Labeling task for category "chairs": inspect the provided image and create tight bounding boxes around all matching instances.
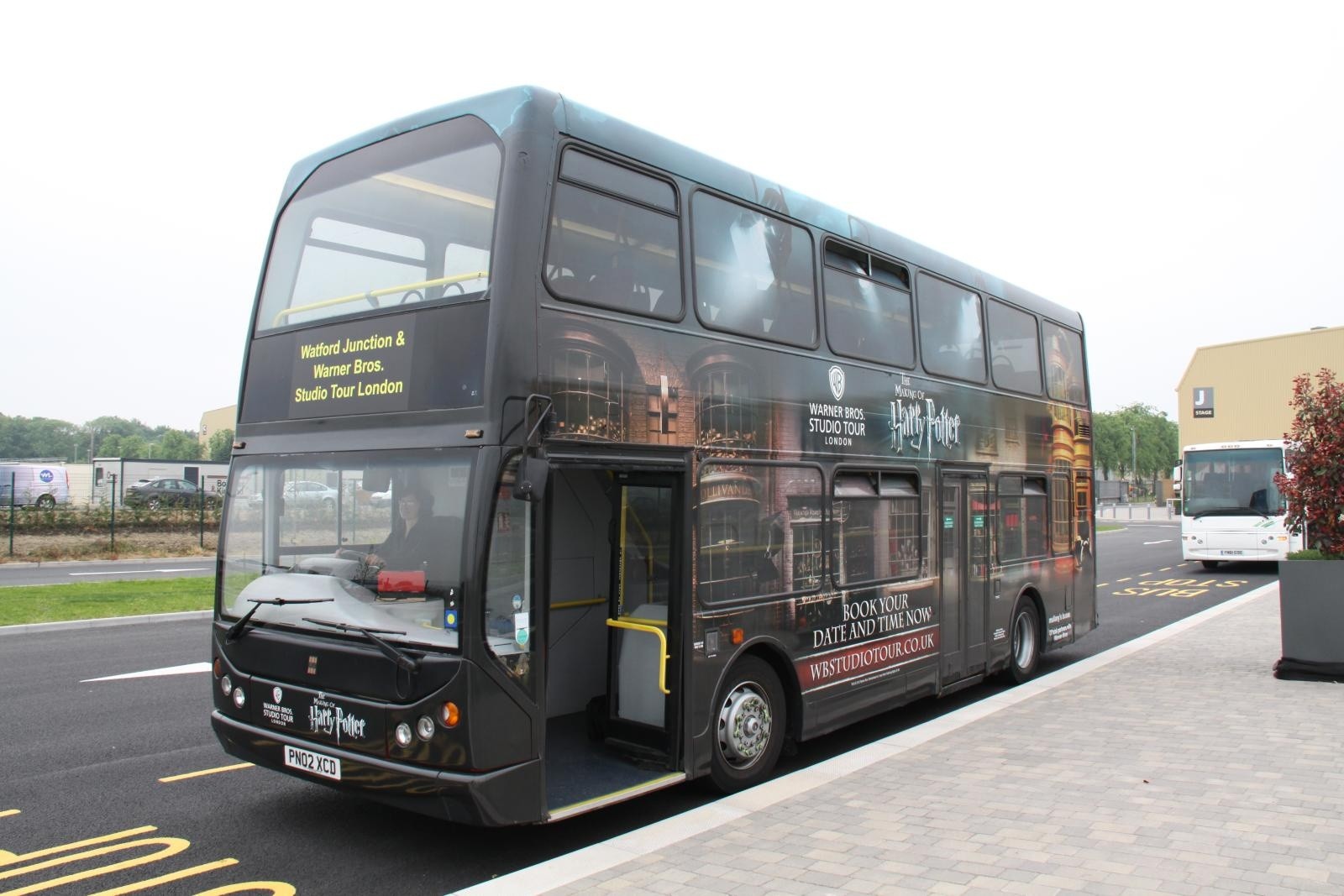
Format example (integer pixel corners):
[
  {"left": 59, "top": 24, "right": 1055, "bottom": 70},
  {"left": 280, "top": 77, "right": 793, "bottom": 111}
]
[{"left": 548, "top": 261, "right": 1085, "bottom": 401}]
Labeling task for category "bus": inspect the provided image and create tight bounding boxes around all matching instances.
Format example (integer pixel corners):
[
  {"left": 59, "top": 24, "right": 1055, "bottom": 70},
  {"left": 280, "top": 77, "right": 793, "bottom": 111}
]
[
  {"left": 212, "top": 86, "right": 1098, "bottom": 825},
  {"left": 1173, "top": 440, "right": 1310, "bottom": 570}
]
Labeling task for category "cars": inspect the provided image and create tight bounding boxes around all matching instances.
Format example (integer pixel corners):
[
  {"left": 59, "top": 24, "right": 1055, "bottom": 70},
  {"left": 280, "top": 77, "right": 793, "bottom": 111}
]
[
  {"left": 250, "top": 480, "right": 346, "bottom": 510},
  {"left": 124, "top": 477, "right": 225, "bottom": 511}
]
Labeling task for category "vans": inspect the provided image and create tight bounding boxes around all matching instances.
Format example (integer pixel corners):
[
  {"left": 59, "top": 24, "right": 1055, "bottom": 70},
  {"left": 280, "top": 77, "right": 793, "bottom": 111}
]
[{"left": 0, "top": 462, "right": 70, "bottom": 511}]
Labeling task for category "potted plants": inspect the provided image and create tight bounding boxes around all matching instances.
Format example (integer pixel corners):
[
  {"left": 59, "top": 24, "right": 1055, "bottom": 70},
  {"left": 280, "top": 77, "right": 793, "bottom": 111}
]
[{"left": 1271, "top": 548, "right": 1344, "bottom": 683}]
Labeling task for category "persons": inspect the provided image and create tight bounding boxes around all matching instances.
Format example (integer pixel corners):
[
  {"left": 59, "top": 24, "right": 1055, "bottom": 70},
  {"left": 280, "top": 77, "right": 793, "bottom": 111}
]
[{"left": 333, "top": 482, "right": 450, "bottom": 572}]
